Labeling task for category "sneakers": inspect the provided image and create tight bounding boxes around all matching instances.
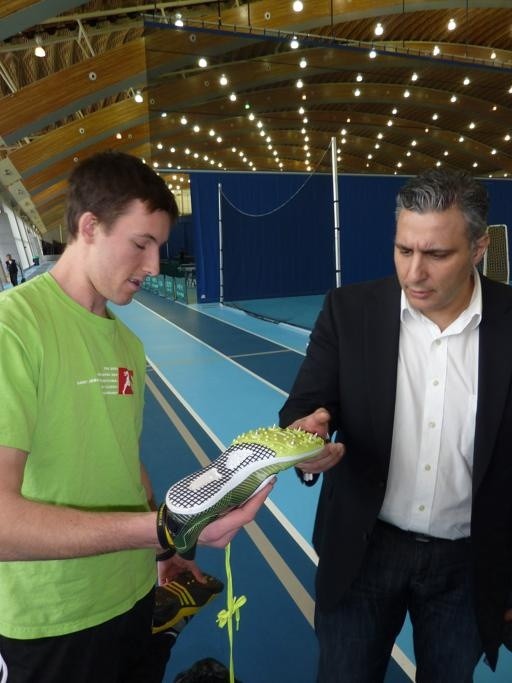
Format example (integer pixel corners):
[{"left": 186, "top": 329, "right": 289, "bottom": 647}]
[
  {"left": 153, "top": 571, "right": 223, "bottom": 633},
  {"left": 162, "top": 427, "right": 324, "bottom": 560}
]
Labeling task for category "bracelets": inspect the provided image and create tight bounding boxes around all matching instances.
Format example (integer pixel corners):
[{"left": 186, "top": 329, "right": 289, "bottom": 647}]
[{"left": 153, "top": 499, "right": 176, "bottom": 550}]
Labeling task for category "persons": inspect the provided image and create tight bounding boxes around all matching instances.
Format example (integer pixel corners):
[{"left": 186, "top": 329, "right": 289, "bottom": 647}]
[
  {"left": 272, "top": 165, "right": 510, "bottom": 682},
  {"left": 0, "top": 142, "right": 280, "bottom": 681},
  {"left": 3, "top": 253, "right": 21, "bottom": 288}
]
